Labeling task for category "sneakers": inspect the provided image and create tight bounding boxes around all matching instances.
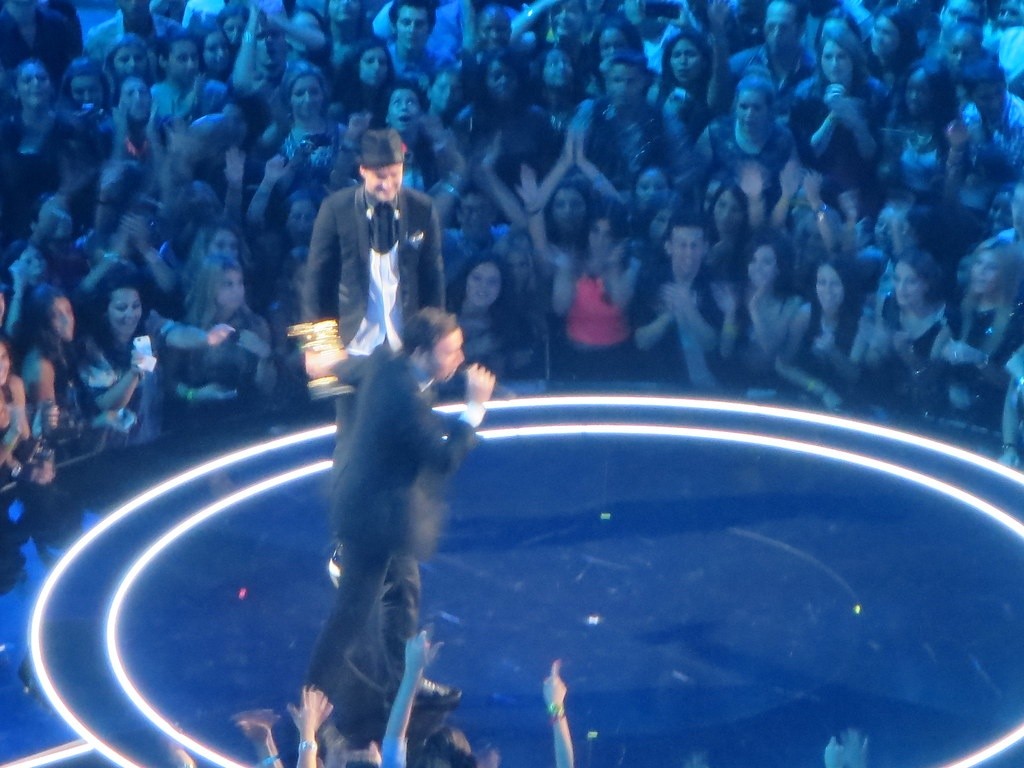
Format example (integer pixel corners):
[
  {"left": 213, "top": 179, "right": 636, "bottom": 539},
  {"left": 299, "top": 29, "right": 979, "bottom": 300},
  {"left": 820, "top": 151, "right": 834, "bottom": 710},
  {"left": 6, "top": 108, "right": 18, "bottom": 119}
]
[
  {"left": 383, "top": 678, "right": 464, "bottom": 711},
  {"left": 330, "top": 542, "right": 343, "bottom": 575}
]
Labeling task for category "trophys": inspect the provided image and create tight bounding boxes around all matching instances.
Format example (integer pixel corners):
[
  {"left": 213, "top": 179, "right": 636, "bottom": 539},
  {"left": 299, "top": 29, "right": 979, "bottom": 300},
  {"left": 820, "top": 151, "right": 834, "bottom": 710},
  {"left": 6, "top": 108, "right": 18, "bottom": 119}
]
[{"left": 285, "top": 318, "right": 353, "bottom": 401}]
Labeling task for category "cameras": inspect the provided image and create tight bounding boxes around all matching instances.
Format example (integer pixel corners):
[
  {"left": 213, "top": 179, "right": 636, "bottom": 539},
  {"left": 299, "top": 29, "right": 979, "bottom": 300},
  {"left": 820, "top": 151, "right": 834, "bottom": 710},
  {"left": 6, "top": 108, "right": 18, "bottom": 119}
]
[{"left": 27, "top": 439, "right": 53, "bottom": 464}]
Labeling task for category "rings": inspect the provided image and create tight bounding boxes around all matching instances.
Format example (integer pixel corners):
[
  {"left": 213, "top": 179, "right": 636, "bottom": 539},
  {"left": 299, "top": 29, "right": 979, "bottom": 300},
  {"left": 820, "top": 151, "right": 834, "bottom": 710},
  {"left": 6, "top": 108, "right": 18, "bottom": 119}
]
[{"left": 955, "top": 352, "right": 957, "bottom": 357}]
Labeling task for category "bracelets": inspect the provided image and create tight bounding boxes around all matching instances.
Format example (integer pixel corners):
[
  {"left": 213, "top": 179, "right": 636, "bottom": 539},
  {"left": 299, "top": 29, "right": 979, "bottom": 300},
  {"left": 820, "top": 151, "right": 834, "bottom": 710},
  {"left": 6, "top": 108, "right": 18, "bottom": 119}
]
[
  {"left": 526, "top": 208, "right": 542, "bottom": 216},
  {"left": 551, "top": 714, "right": 565, "bottom": 721},
  {"left": 299, "top": 741, "right": 317, "bottom": 751},
  {"left": 186, "top": 388, "right": 195, "bottom": 400},
  {"left": 976, "top": 354, "right": 989, "bottom": 369},
  {"left": 723, "top": 324, "right": 737, "bottom": 335},
  {"left": 262, "top": 754, "right": 280, "bottom": 768},
  {"left": 1001, "top": 442, "right": 1017, "bottom": 449},
  {"left": 813, "top": 204, "right": 828, "bottom": 221},
  {"left": 807, "top": 380, "right": 816, "bottom": 392}
]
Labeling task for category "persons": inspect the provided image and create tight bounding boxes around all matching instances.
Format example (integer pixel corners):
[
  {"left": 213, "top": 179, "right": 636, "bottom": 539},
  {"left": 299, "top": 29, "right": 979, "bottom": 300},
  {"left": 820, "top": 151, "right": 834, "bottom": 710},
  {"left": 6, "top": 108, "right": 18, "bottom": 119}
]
[
  {"left": 302, "top": 126, "right": 444, "bottom": 586},
  {"left": 235, "top": 630, "right": 574, "bottom": 768},
  {"left": 824, "top": 728, "right": 869, "bottom": 768},
  {"left": 0, "top": 0, "right": 1024, "bottom": 485},
  {"left": 308, "top": 307, "right": 497, "bottom": 723}
]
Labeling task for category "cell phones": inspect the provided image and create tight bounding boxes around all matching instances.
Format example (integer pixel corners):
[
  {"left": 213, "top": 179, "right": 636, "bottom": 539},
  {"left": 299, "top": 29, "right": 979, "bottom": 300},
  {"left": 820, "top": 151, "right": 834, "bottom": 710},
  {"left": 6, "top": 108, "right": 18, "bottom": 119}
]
[
  {"left": 308, "top": 132, "right": 334, "bottom": 146},
  {"left": 132, "top": 334, "right": 152, "bottom": 357}
]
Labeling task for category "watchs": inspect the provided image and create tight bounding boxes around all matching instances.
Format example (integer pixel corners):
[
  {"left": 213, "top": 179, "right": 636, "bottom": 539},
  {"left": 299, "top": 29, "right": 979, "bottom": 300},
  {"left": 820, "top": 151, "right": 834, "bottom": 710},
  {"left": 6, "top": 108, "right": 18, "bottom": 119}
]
[{"left": 548, "top": 704, "right": 563, "bottom": 714}]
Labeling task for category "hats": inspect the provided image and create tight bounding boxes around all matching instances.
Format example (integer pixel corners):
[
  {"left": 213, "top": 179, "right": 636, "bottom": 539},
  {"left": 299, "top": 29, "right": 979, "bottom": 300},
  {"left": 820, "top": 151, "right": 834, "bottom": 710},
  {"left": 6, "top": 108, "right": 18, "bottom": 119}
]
[{"left": 355, "top": 128, "right": 406, "bottom": 169}]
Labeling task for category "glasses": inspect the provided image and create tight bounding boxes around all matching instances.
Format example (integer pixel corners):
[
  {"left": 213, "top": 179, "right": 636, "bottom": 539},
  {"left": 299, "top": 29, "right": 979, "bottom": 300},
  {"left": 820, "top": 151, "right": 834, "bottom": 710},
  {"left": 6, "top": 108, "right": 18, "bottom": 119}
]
[{"left": 256, "top": 29, "right": 281, "bottom": 41}]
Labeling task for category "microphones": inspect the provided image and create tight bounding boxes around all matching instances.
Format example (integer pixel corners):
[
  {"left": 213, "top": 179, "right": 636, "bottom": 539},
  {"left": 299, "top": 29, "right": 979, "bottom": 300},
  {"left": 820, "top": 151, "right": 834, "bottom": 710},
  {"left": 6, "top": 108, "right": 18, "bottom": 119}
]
[{"left": 460, "top": 365, "right": 512, "bottom": 399}]
